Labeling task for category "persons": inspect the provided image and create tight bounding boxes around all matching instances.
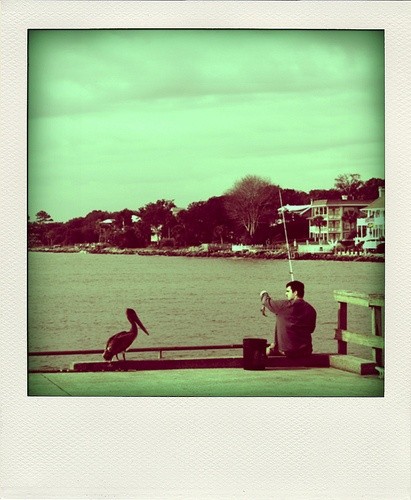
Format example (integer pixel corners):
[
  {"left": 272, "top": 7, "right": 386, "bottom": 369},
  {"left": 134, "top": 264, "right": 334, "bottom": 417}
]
[{"left": 259, "top": 281, "right": 317, "bottom": 356}]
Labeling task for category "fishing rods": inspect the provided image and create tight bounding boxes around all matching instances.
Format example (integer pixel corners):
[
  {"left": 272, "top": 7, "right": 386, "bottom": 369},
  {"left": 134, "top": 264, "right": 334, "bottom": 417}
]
[{"left": 279, "top": 188, "right": 294, "bottom": 282}]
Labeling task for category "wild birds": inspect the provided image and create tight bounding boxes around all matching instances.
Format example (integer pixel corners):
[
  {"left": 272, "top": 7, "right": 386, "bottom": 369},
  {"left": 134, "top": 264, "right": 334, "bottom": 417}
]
[{"left": 103, "top": 308, "right": 150, "bottom": 372}]
